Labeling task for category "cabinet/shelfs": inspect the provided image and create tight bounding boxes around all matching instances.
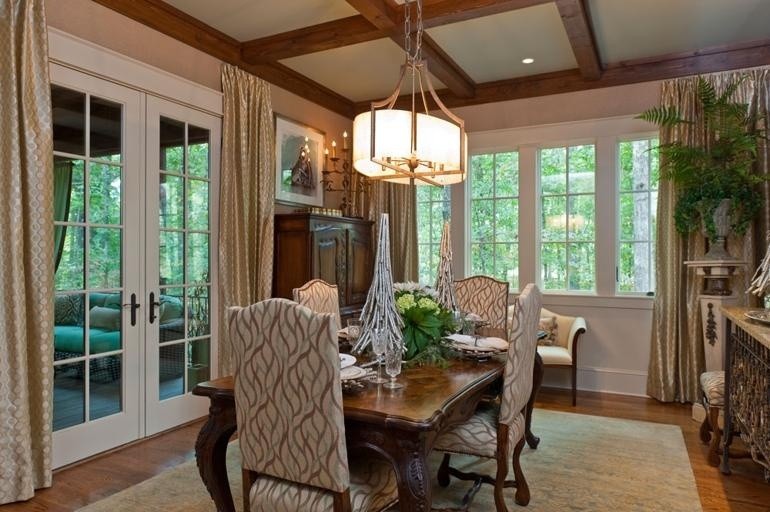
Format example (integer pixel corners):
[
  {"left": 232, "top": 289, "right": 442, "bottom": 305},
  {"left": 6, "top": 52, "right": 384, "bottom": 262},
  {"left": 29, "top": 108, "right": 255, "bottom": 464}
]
[{"left": 269, "top": 214, "right": 375, "bottom": 325}]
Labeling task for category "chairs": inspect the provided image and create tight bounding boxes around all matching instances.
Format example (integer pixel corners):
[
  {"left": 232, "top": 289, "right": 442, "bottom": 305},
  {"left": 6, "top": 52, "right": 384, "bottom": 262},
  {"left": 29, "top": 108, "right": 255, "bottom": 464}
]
[
  {"left": 223, "top": 297, "right": 400, "bottom": 512},
  {"left": 429, "top": 282, "right": 542, "bottom": 512},
  {"left": 446, "top": 273, "right": 510, "bottom": 343},
  {"left": 507, "top": 304, "right": 588, "bottom": 408},
  {"left": 292, "top": 276, "right": 341, "bottom": 350}
]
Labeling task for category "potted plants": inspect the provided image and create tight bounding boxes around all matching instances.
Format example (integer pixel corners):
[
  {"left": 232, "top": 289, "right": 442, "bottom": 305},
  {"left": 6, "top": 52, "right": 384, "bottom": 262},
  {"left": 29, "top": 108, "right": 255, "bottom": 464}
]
[{"left": 630, "top": 70, "right": 770, "bottom": 264}]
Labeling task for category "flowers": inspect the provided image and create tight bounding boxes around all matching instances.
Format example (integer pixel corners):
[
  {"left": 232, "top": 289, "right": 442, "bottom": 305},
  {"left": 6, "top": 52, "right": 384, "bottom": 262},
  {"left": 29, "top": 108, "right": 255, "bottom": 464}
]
[{"left": 391, "top": 275, "right": 450, "bottom": 357}]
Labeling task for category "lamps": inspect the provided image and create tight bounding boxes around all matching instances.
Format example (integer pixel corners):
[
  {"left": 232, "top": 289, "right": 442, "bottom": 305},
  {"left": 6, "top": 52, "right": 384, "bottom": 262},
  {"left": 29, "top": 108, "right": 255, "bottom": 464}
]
[
  {"left": 320, "top": 129, "right": 369, "bottom": 217},
  {"left": 351, "top": 0, "right": 470, "bottom": 189}
]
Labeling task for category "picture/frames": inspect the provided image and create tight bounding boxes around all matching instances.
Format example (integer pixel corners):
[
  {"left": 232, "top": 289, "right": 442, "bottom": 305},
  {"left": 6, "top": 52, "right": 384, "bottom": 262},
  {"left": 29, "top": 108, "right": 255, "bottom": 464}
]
[{"left": 274, "top": 111, "right": 326, "bottom": 212}]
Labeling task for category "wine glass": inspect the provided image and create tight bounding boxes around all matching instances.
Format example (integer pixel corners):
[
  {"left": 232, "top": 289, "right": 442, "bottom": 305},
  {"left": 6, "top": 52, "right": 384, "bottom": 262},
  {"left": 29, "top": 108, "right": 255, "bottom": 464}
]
[
  {"left": 370, "top": 328, "right": 390, "bottom": 384},
  {"left": 384, "top": 350, "right": 402, "bottom": 391},
  {"left": 449, "top": 310, "right": 464, "bottom": 342},
  {"left": 347, "top": 317, "right": 362, "bottom": 363}
]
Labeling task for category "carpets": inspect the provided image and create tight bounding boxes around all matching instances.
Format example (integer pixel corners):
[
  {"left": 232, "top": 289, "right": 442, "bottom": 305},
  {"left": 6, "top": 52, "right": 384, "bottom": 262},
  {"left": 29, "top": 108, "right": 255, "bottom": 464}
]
[{"left": 72, "top": 406, "right": 708, "bottom": 512}]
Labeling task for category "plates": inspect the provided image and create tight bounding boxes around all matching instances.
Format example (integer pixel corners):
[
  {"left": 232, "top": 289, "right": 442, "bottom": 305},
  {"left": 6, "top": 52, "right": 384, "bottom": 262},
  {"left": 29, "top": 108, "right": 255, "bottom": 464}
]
[
  {"left": 339, "top": 353, "right": 354, "bottom": 370},
  {"left": 340, "top": 366, "right": 365, "bottom": 380}
]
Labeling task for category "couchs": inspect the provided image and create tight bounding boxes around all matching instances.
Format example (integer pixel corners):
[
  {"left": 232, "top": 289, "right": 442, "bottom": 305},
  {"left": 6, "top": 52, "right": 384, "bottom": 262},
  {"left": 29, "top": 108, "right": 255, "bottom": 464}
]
[{"left": 49, "top": 289, "right": 195, "bottom": 391}]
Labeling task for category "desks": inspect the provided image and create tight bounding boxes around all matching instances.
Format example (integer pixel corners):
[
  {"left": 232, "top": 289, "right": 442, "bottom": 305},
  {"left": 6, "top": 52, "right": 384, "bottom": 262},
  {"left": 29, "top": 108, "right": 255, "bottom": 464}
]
[{"left": 720, "top": 305, "right": 770, "bottom": 481}]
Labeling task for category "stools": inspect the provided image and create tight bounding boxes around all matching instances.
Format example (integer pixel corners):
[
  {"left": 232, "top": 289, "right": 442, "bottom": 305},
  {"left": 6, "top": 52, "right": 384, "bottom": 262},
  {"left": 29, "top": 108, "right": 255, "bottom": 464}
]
[{"left": 697, "top": 370, "right": 727, "bottom": 466}]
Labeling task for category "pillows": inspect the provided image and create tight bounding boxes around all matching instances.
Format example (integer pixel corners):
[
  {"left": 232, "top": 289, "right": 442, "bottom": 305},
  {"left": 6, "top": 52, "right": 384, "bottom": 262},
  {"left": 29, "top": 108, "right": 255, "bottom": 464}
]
[
  {"left": 55, "top": 290, "right": 83, "bottom": 327},
  {"left": 538, "top": 314, "right": 557, "bottom": 346},
  {"left": 88, "top": 305, "right": 124, "bottom": 333}
]
